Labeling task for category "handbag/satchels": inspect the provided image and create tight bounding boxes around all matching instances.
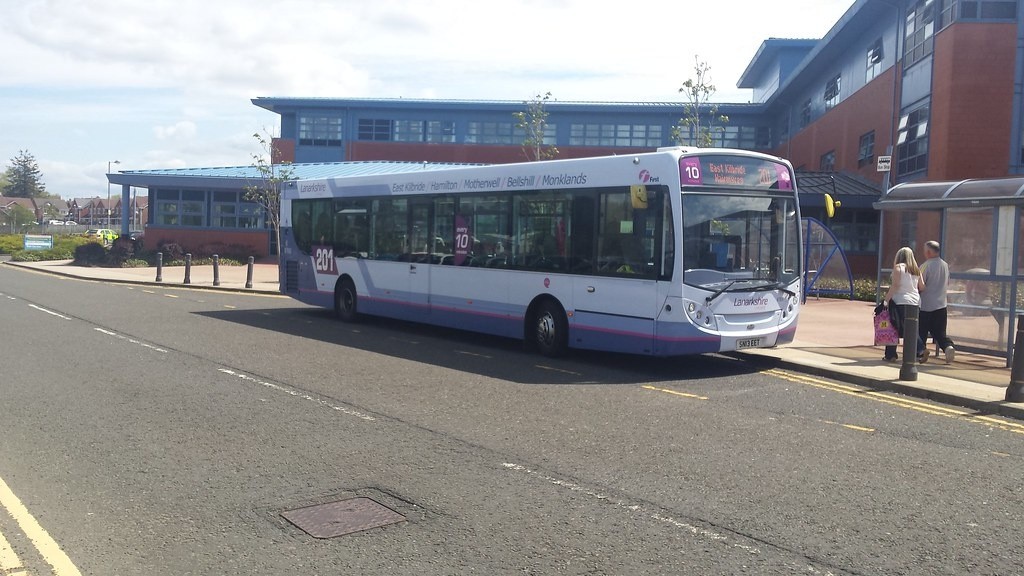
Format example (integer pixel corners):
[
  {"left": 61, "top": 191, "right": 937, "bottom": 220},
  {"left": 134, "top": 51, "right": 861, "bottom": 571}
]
[{"left": 874, "top": 309, "right": 899, "bottom": 346}]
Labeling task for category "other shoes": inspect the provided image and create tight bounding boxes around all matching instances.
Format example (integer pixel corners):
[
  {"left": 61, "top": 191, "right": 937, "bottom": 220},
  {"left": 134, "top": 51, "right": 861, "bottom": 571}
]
[
  {"left": 882, "top": 356, "right": 896, "bottom": 362},
  {"left": 945, "top": 346, "right": 954, "bottom": 363},
  {"left": 919, "top": 348, "right": 930, "bottom": 364}
]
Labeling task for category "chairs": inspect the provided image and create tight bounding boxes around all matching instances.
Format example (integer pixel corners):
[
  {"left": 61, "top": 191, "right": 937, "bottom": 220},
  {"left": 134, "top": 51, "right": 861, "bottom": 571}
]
[{"left": 397, "top": 249, "right": 528, "bottom": 269}]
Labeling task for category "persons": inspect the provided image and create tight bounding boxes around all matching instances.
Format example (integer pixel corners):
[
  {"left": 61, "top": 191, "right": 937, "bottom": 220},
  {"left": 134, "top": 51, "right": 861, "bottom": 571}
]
[
  {"left": 882, "top": 246, "right": 929, "bottom": 364},
  {"left": 918, "top": 240, "right": 955, "bottom": 363}
]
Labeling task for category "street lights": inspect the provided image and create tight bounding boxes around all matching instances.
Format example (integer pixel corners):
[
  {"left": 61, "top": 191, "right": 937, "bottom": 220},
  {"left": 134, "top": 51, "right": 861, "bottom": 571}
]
[{"left": 108, "top": 160, "right": 121, "bottom": 229}]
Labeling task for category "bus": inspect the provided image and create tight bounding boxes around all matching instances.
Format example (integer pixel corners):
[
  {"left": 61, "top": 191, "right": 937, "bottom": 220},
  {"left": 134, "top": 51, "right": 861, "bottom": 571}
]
[{"left": 278, "top": 145, "right": 841, "bottom": 358}]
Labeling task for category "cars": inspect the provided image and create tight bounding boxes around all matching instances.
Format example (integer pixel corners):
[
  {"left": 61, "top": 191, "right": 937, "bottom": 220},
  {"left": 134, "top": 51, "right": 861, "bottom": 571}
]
[
  {"left": 50, "top": 220, "right": 64, "bottom": 225},
  {"left": 84, "top": 229, "right": 118, "bottom": 245},
  {"left": 66, "top": 221, "right": 77, "bottom": 225}
]
